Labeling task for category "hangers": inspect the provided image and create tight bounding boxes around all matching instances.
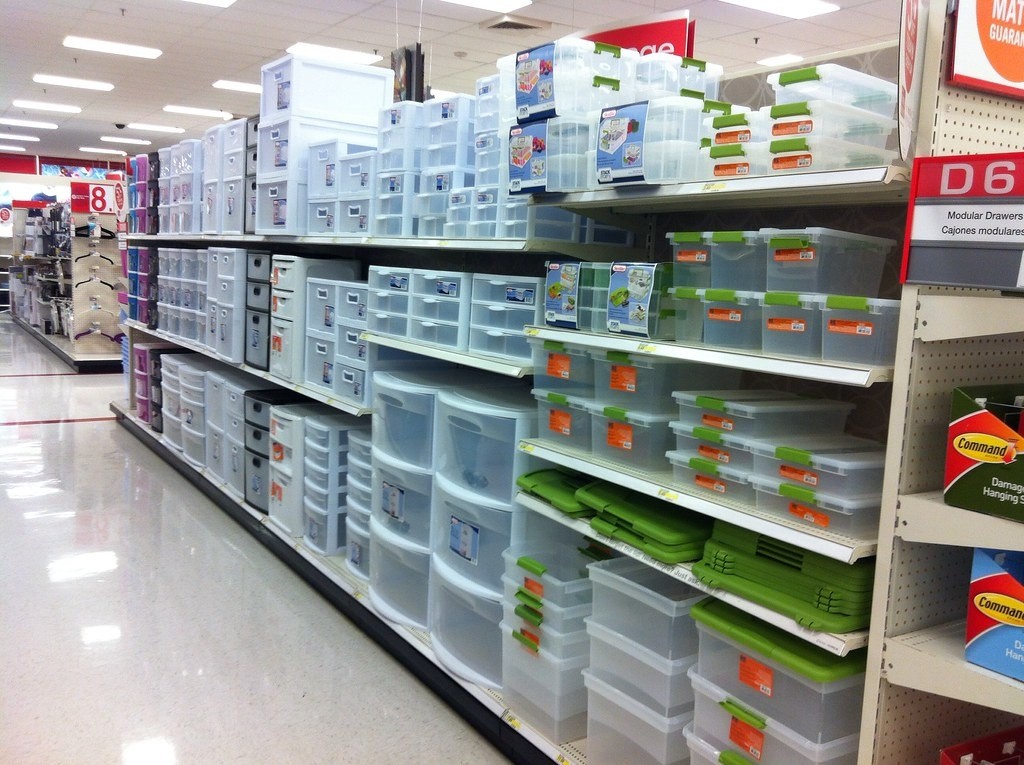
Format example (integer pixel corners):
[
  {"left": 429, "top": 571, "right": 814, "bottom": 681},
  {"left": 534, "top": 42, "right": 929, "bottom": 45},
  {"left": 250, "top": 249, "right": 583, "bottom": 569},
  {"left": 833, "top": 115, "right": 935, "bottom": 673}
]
[
  {"left": 75, "top": 269, "right": 115, "bottom": 291},
  {"left": 79, "top": 298, "right": 115, "bottom": 317},
  {"left": 75, "top": 325, "right": 115, "bottom": 342},
  {"left": 68, "top": 225, "right": 115, "bottom": 240},
  {"left": 75, "top": 254, "right": 114, "bottom": 264}
]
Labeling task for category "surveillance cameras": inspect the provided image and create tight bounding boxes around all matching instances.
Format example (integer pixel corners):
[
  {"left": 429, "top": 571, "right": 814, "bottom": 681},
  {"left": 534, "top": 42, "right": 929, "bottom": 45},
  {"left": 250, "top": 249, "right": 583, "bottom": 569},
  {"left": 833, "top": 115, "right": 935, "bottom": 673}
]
[{"left": 115, "top": 124, "right": 125, "bottom": 130}]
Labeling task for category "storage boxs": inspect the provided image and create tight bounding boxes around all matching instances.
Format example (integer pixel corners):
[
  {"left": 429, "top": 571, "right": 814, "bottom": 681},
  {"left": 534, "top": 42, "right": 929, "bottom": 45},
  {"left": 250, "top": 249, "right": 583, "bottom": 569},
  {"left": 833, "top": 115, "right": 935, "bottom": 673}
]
[
  {"left": 121, "top": 37, "right": 899, "bottom": 765},
  {"left": 965, "top": 548, "right": 1024, "bottom": 683},
  {"left": 943, "top": 382, "right": 1024, "bottom": 523},
  {"left": 939, "top": 726, "right": 1024, "bottom": 765}
]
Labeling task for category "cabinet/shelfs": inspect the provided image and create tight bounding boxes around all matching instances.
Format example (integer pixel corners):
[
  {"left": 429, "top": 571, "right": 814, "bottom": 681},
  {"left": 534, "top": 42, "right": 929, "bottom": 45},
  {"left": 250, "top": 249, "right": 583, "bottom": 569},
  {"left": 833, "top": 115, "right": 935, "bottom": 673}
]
[
  {"left": 9, "top": 208, "right": 123, "bottom": 373},
  {"left": 858, "top": 278, "right": 1024, "bottom": 765},
  {"left": 517, "top": 166, "right": 909, "bottom": 657},
  {"left": 111, "top": 232, "right": 654, "bottom": 765}
]
